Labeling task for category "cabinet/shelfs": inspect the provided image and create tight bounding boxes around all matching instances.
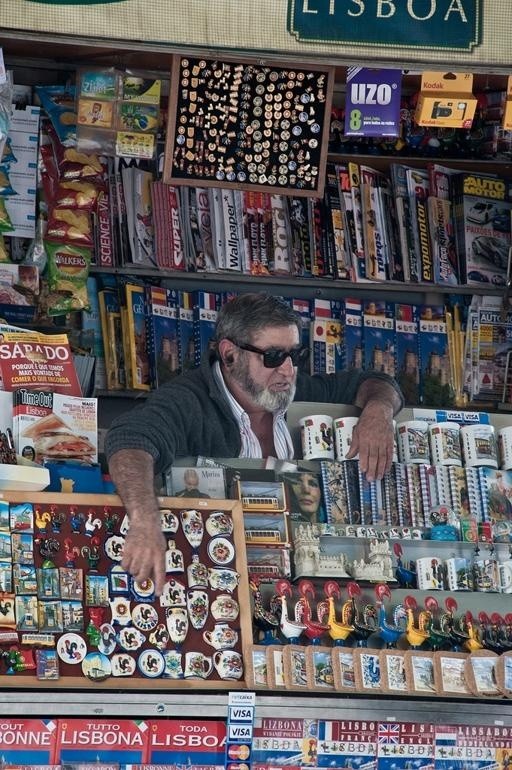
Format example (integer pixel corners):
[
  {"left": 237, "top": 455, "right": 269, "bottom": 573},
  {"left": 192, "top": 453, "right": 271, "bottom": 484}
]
[
  {"left": 0, "top": 29, "right": 512, "bottom": 413},
  {"left": 0, "top": 691, "right": 512, "bottom": 770}
]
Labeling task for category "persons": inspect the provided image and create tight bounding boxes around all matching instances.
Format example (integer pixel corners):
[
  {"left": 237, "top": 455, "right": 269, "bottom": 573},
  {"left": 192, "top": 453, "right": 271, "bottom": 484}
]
[{"left": 104, "top": 292, "right": 406, "bottom": 597}]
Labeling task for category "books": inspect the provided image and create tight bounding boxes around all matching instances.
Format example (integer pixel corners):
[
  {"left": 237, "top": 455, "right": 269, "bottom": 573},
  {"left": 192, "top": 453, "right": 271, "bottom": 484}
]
[
  {"left": 98, "top": 290, "right": 125, "bottom": 390},
  {"left": 193, "top": 290, "right": 221, "bottom": 368},
  {"left": 0, "top": 717, "right": 510, "bottom": 764},
  {"left": 285, "top": 296, "right": 311, "bottom": 347},
  {"left": 95, "top": 155, "right": 512, "bottom": 285},
  {"left": 144, "top": 287, "right": 179, "bottom": 390},
  {"left": 224, "top": 291, "right": 242, "bottom": 304},
  {"left": 390, "top": 303, "right": 420, "bottom": 381},
  {"left": 361, "top": 299, "right": 395, "bottom": 376},
  {"left": 118, "top": 285, "right": 151, "bottom": 391},
  {"left": 450, "top": 294, "right": 512, "bottom": 404},
  {"left": 320, "top": 458, "right": 512, "bottom": 529},
  {"left": 309, "top": 297, "right": 344, "bottom": 376},
  {"left": 82, "top": 277, "right": 105, "bottom": 389},
  {"left": 14, "top": 391, "right": 96, "bottom": 466},
  {"left": 176, "top": 290, "right": 194, "bottom": 375},
  {"left": 341, "top": 297, "right": 363, "bottom": 370},
  {"left": 417, "top": 305, "right": 448, "bottom": 405},
  {"left": 0, "top": 331, "right": 83, "bottom": 398}
]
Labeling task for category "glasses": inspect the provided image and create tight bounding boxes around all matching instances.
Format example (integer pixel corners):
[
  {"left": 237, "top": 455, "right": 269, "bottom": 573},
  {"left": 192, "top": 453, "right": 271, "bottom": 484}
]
[{"left": 233, "top": 340, "right": 310, "bottom": 366}]
